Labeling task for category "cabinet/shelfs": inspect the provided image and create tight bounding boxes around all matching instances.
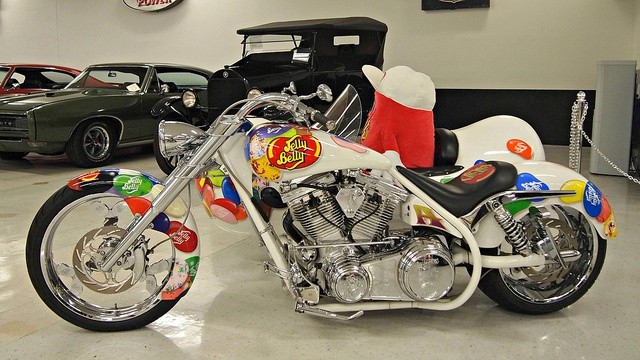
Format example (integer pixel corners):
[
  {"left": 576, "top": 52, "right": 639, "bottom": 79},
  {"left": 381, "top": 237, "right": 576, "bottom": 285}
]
[{"left": 589, "top": 58, "right": 635, "bottom": 176}]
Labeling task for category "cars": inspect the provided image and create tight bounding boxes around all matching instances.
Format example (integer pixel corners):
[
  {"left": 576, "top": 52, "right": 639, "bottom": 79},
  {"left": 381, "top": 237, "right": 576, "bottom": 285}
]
[
  {"left": 0, "top": 62, "right": 214, "bottom": 168},
  {"left": 0, "top": 64, "right": 122, "bottom": 155}
]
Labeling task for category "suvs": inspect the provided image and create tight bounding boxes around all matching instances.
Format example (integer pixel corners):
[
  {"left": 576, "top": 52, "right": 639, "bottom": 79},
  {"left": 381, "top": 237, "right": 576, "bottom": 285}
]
[{"left": 150, "top": 17, "right": 388, "bottom": 175}]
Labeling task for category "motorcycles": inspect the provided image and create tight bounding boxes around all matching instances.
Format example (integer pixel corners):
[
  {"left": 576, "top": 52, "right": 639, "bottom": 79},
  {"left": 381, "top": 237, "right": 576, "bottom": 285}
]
[{"left": 25, "top": 81, "right": 618, "bottom": 332}]
